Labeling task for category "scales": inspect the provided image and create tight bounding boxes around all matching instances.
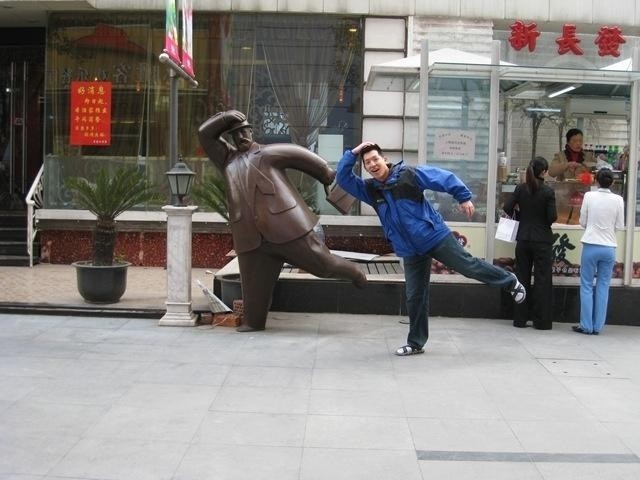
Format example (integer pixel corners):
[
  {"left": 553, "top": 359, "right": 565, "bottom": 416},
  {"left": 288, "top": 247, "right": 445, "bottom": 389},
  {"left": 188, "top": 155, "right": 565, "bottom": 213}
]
[{"left": 595, "top": 170, "right": 623, "bottom": 179}]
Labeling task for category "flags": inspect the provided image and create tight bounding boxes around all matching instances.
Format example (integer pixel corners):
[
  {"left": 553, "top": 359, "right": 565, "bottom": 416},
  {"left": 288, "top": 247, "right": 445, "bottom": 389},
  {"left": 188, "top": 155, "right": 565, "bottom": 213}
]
[
  {"left": 180, "top": 0, "right": 196, "bottom": 79},
  {"left": 158, "top": 0, "right": 182, "bottom": 64}
]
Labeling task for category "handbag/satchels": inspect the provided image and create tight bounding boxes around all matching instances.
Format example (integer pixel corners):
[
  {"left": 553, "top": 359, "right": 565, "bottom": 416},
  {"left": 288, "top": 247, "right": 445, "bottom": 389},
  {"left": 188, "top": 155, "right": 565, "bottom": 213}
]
[{"left": 494, "top": 208, "right": 520, "bottom": 244}]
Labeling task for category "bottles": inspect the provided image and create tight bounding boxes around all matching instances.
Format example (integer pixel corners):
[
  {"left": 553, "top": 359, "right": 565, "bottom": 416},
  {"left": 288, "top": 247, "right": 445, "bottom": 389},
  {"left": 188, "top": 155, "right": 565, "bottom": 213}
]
[
  {"left": 497, "top": 152, "right": 507, "bottom": 182},
  {"left": 584, "top": 143, "right": 619, "bottom": 169}
]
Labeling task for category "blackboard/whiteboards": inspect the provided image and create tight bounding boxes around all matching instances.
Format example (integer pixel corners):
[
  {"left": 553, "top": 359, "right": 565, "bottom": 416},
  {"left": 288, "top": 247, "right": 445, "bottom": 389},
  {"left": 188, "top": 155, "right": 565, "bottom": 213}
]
[{"left": 69, "top": 81, "right": 113, "bottom": 146}]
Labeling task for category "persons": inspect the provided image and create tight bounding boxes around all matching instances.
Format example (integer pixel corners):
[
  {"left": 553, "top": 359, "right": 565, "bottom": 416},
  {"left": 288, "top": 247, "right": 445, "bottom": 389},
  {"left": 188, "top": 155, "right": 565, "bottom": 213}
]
[
  {"left": 595, "top": 144, "right": 631, "bottom": 195},
  {"left": 505, "top": 155, "right": 555, "bottom": 332},
  {"left": 570, "top": 167, "right": 625, "bottom": 336},
  {"left": 336, "top": 138, "right": 527, "bottom": 358},
  {"left": 547, "top": 127, "right": 607, "bottom": 225},
  {"left": 196, "top": 108, "right": 366, "bottom": 333}
]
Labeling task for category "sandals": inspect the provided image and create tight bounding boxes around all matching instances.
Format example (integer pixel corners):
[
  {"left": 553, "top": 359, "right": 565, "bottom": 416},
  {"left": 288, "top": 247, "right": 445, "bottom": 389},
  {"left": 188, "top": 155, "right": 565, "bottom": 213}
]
[
  {"left": 509, "top": 273, "right": 526, "bottom": 304},
  {"left": 395, "top": 345, "right": 424, "bottom": 356}
]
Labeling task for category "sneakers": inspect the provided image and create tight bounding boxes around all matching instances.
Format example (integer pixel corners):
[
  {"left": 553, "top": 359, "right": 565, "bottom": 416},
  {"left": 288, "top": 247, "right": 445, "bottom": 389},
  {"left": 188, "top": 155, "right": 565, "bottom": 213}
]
[{"left": 572, "top": 326, "right": 600, "bottom": 336}]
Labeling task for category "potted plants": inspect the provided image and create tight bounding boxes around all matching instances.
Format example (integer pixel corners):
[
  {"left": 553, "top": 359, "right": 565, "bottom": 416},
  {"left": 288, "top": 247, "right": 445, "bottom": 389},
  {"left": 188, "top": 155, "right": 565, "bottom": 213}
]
[{"left": 64, "top": 163, "right": 158, "bottom": 304}]
